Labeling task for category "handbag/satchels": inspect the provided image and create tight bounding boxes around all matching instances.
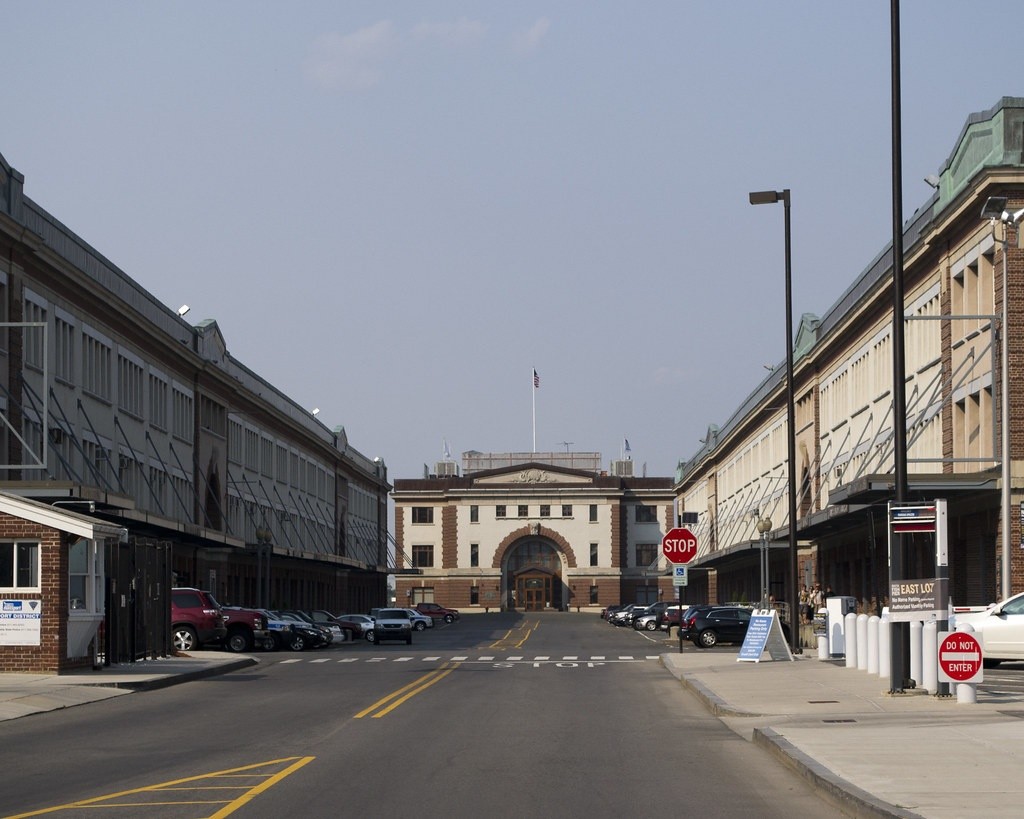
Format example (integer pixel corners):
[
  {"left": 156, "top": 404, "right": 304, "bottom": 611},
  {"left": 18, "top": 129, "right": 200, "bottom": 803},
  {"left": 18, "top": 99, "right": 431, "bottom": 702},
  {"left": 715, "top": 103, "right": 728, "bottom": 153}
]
[{"left": 810, "top": 603, "right": 815, "bottom": 608}]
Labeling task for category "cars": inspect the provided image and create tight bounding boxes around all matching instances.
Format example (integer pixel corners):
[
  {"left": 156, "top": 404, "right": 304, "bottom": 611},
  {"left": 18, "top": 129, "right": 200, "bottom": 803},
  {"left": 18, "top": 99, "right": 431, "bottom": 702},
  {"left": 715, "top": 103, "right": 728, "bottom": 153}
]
[
  {"left": 677, "top": 605, "right": 724, "bottom": 641},
  {"left": 626, "top": 602, "right": 689, "bottom": 626},
  {"left": 371, "top": 608, "right": 433, "bottom": 632},
  {"left": 601, "top": 604, "right": 654, "bottom": 627},
  {"left": 636, "top": 615, "right": 669, "bottom": 631},
  {"left": 257, "top": 610, "right": 342, "bottom": 652},
  {"left": 374, "top": 608, "right": 413, "bottom": 646},
  {"left": 948, "top": 592, "right": 1024, "bottom": 666},
  {"left": 305, "top": 610, "right": 362, "bottom": 643},
  {"left": 663, "top": 605, "right": 689, "bottom": 625},
  {"left": 416, "top": 603, "right": 459, "bottom": 624},
  {"left": 337, "top": 614, "right": 376, "bottom": 643},
  {"left": 688, "top": 608, "right": 791, "bottom": 648}
]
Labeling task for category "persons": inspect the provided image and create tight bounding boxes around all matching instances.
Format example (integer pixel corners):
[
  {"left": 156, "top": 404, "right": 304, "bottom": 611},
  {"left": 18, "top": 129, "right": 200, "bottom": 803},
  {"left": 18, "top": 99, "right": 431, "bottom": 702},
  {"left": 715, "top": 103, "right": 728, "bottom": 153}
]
[
  {"left": 764, "top": 589, "right": 775, "bottom": 602},
  {"left": 798, "top": 583, "right": 835, "bottom": 625}
]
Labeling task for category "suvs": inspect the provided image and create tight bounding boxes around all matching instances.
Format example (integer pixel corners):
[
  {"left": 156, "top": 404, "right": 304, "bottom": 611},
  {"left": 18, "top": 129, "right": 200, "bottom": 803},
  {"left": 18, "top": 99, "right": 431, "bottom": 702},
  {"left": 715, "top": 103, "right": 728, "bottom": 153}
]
[{"left": 169, "top": 588, "right": 270, "bottom": 654}]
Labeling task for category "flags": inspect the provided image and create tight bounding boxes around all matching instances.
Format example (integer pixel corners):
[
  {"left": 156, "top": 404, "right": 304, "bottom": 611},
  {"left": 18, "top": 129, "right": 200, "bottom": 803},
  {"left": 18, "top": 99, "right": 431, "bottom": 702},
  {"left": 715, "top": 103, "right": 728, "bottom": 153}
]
[
  {"left": 624, "top": 437, "right": 631, "bottom": 452},
  {"left": 533, "top": 367, "right": 540, "bottom": 388}
]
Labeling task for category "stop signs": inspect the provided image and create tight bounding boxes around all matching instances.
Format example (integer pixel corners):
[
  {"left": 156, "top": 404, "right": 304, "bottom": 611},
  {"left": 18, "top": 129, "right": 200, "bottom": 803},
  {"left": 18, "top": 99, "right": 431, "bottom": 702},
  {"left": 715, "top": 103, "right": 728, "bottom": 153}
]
[
  {"left": 937, "top": 632, "right": 984, "bottom": 683},
  {"left": 662, "top": 528, "right": 698, "bottom": 564}
]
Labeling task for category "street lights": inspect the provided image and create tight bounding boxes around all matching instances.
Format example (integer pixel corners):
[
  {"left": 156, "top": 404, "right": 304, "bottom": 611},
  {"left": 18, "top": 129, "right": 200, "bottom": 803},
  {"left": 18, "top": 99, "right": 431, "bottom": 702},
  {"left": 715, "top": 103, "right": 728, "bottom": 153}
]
[
  {"left": 757, "top": 514, "right": 773, "bottom": 613},
  {"left": 749, "top": 187, "right": 799, "bottom": 656}
]
